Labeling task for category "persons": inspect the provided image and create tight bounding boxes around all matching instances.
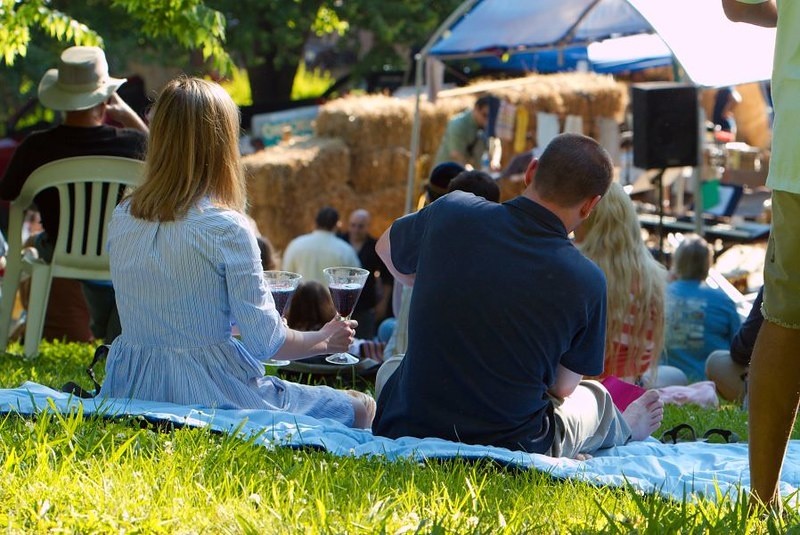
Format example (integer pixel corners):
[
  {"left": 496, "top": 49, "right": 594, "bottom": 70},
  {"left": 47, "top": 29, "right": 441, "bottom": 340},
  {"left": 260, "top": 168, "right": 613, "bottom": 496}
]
[
  {"left": 722, "top": 0, "right": 800, "bottom": 518},
  {"left": 0, "top": 48, "right": 766, "bottom": 459},
  {"left": 370, "top": 133, "right": 664, "bottom": 460},
  {"left": 94, "top": 75, "right": 377, "bottom": 428}
]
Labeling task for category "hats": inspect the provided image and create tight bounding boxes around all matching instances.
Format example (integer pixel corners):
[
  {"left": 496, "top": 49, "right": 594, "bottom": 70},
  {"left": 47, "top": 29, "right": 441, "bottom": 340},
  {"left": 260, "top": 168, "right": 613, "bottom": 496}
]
[{"left": 37, "top": 45, "right": 129, "bottom": 111}]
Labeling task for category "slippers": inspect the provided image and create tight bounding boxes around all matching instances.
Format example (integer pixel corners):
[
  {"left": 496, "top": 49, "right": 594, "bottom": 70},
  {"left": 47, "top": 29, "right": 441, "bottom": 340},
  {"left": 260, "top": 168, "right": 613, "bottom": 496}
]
[
  {"left": 657, "top": 424, "right": 709, "bottom": 445},
  {"left": 703, "top": 428, "right": 739, "bottom": 444}
]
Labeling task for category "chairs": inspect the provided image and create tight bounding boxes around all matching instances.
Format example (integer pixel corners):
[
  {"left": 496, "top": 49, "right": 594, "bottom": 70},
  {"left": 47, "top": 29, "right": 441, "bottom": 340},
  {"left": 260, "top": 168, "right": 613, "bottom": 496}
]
[{"left": 0, "top": 156, "right": 147, "bottom": 360}]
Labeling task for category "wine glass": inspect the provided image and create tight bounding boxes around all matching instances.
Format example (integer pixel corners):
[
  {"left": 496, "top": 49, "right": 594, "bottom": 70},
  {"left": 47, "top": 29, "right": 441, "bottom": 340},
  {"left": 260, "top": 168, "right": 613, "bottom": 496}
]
[
  {"left": 260, "top": 270, "right": 303, "bottom": 366},
  {"left": 322, "top": 266, "right": 370, "bottom": 365}
]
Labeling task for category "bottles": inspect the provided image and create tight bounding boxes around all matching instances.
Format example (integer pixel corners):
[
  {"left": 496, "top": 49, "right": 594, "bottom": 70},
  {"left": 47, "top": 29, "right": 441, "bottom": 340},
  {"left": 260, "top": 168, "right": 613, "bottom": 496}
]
[{"left": 481, "top": 150, "right": 490, "bottom": 174}]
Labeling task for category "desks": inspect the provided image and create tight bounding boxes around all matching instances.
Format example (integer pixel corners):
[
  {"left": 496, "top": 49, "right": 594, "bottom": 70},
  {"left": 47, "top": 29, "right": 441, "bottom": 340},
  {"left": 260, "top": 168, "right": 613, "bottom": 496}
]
[{"left": 640, "top": 213, "right": 771, "bottom": 264}]
